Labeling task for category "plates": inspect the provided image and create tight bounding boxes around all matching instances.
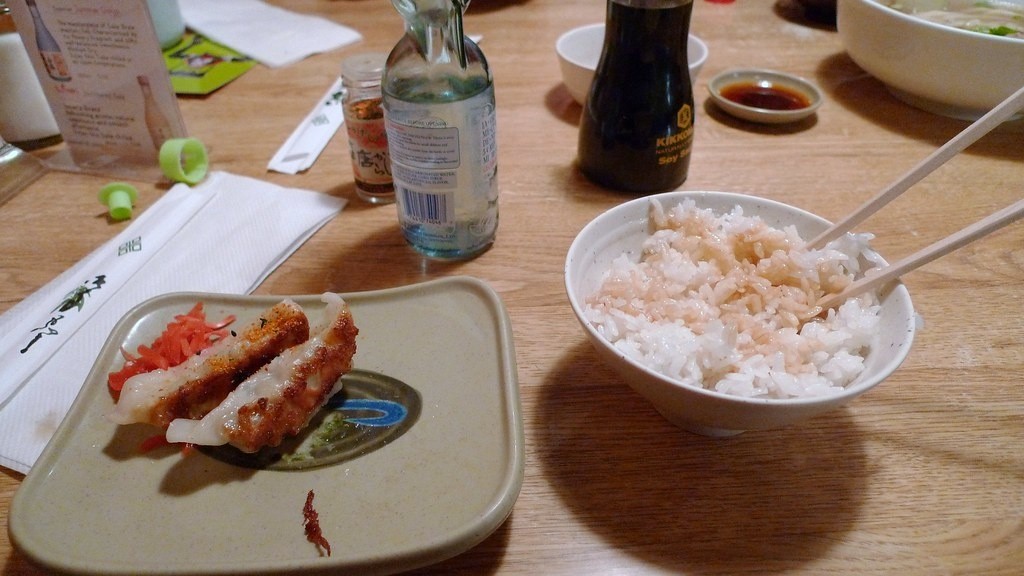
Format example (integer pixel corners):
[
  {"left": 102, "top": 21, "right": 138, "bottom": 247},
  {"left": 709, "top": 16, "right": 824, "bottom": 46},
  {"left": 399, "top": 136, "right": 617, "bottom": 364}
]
[
  {"left": 7, "top": 274, "right": 525, "bottom": 576},
  {"left": 707, "top": 69, "right": 826, "bottom": 125}
]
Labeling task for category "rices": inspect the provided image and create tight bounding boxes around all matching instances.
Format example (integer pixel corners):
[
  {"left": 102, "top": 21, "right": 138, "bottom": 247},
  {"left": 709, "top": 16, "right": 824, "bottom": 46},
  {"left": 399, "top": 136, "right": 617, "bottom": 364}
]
[{"left": 582, "top": 196, "right": 890, "bottom": 400}]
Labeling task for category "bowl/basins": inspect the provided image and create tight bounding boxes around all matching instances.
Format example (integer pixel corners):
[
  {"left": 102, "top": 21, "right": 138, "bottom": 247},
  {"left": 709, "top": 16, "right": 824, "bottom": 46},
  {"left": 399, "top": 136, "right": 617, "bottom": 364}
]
[
  {"left": 836, "top": 0, "right": 1024, "bottom": 122},
  {"left": 563, "top": 190, "right": 916, "bottom": 438},
  {"left": 556, "top": 23, "right": 709, "bottom": 107}
]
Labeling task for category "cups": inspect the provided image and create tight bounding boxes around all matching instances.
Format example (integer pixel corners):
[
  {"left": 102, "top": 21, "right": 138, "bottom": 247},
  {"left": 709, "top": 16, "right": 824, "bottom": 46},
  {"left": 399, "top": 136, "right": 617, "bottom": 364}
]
[{"left": 0, "top": 6, "right": 64, "bottom": 151}]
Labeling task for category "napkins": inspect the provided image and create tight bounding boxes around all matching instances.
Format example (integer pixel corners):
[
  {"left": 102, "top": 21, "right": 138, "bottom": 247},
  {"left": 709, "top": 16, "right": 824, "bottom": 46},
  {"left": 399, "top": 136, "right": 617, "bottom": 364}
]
[{"left": 179, "top": 0, "right": 363, "bottom": 68}]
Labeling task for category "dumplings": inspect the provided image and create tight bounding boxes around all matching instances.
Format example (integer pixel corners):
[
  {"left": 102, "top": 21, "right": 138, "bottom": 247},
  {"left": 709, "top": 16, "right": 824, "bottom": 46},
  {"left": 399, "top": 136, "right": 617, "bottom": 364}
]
[
  {"left": 107, "top": 296, "right": 310, "bottom": 429},
  {"left": 166, "top": 292, "right": 360, "bottom": 453}
]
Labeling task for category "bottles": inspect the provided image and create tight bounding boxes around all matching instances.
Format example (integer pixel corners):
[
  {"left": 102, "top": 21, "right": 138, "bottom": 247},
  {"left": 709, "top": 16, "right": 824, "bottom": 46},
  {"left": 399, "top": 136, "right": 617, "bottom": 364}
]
[
  {"left": 577, "top": 0, "right": 695, "bottom": 198},
  {"left": 380, "top": 0, "right": 501, "bottom": 263},
  {"left": 340, "top": 52, "right": 396, "bottom": 204}
]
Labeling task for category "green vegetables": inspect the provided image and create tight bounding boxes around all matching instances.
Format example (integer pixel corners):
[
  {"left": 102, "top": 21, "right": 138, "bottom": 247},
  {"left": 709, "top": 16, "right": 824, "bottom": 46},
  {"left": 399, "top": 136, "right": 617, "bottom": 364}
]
[{"left": 960, "top": 0, "right": 1024, "bottom": 38}]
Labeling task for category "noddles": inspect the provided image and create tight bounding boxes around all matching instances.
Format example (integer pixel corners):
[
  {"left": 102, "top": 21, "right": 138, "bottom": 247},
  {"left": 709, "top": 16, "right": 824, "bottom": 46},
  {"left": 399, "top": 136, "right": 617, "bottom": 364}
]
[{"left": 879, "top": 0, "right": 1024, "bottom": 41}]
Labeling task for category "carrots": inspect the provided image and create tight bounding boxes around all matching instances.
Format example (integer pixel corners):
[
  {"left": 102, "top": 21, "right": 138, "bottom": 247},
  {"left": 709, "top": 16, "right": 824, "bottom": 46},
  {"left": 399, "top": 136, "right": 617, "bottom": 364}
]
[{"left": 109, "top": 301, "right": 237, "bottom": 454}]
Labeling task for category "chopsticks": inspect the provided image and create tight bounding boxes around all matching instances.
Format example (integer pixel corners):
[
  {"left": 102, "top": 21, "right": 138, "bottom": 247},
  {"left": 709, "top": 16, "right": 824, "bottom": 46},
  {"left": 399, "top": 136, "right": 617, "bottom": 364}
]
[{"left": 799, "top": 86, "right": 1024, "bottom": 313}]
